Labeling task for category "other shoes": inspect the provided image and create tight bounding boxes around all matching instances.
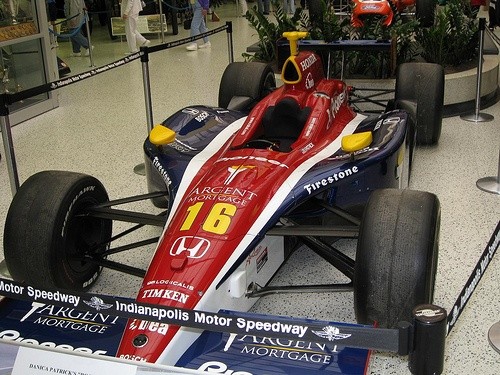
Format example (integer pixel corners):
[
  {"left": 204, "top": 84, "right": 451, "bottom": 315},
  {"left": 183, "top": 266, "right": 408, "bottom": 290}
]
[
  {"left": 85, "top": 46, "right": 95, "bottom": 56},
  {"left": 185, "top": 43, "right": 197, "bottom": 50},
  {"left": 143, "top": 40, "right": 150, "bottom": 46},
  {"left": 69, "top": 52, "right": 81, "bottom": 57},
  {"left": 125, "top": 51, "right": 136, "bottom": 54},
  {"left": 198, "top": 41, "right": 212, "bottom": 49}
]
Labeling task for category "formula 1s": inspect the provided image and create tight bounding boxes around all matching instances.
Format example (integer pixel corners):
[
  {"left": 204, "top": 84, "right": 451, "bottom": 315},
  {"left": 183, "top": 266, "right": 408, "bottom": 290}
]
[{"left": 0, "top": 32, "right": 448, "bottom": 375}]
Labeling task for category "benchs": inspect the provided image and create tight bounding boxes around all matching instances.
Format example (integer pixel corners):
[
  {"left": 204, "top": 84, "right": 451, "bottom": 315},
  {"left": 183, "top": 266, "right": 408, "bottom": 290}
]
[{"left": 110, "top": 14, "right": 167, "bottom": 43}]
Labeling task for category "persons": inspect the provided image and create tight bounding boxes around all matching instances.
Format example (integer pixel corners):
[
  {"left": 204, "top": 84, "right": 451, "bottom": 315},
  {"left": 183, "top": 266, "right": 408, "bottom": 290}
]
[
  {"left": 186, "top": 0, "right": 211, "bottom": 51},
  {"left": 64, "top": 0, "right": 95, "bottom": 57},
  {"left": 120, "top": 0, "right": 150, "bottom": 55}
]
